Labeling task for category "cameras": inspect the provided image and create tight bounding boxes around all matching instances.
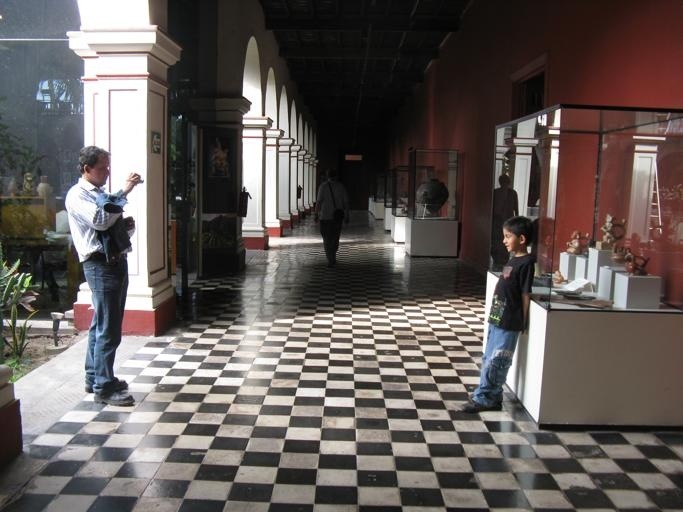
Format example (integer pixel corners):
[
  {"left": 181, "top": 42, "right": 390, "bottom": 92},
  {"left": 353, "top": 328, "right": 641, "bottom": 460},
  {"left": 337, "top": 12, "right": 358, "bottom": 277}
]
[{"left": 138, "top": 175, "right": 144, "bottom": 183}]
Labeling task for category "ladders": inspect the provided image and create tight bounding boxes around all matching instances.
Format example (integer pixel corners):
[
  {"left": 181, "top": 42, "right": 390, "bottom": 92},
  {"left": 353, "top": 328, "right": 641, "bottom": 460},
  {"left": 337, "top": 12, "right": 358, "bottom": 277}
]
[{"left": 648, "top": 162, "right": 663, "bottom": 243}]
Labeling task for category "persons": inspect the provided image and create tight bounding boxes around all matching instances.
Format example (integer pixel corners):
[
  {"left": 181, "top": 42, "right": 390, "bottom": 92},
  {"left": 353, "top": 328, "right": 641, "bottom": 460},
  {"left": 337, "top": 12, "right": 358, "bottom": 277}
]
[
  {"left": 490, "top": 175, "right": 518, "bottom": 264},
  {"left": 464, "top": 216, "right": 535, "bottom": 413},
  {"left": 314, "top": 169, "right": 350, "bottom": 264},
  {"left": 65, "top": 145, "right": 141, "bottom": 406}
]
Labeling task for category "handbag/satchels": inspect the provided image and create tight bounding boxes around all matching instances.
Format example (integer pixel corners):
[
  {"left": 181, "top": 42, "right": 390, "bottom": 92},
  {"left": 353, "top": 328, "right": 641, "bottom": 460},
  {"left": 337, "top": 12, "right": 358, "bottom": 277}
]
[{"left": 333, "top": 209, "right": 344, "bottom": 222}]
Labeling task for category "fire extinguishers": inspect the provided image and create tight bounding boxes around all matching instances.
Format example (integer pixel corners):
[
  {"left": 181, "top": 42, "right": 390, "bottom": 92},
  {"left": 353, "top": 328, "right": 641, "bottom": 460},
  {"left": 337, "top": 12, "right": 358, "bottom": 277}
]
[
  {"left": 238, "top": 186, "right": 252, "bottom": 217},
  {"left": 298, "top": 185, "right": 302, "bottom": 198}
]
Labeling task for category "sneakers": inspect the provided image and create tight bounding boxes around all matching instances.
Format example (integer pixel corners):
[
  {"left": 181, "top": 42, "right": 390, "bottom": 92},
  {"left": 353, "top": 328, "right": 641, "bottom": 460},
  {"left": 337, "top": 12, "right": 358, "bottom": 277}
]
[{"left": 462, "top": 394, "right": 502, "bottom": 413}]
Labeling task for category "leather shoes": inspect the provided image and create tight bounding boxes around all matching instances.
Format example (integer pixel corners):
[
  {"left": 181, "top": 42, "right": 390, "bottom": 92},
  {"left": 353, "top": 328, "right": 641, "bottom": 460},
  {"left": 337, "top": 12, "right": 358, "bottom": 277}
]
[{"left": 85, "top": 379, "right": 135, "bottom": 406}]
